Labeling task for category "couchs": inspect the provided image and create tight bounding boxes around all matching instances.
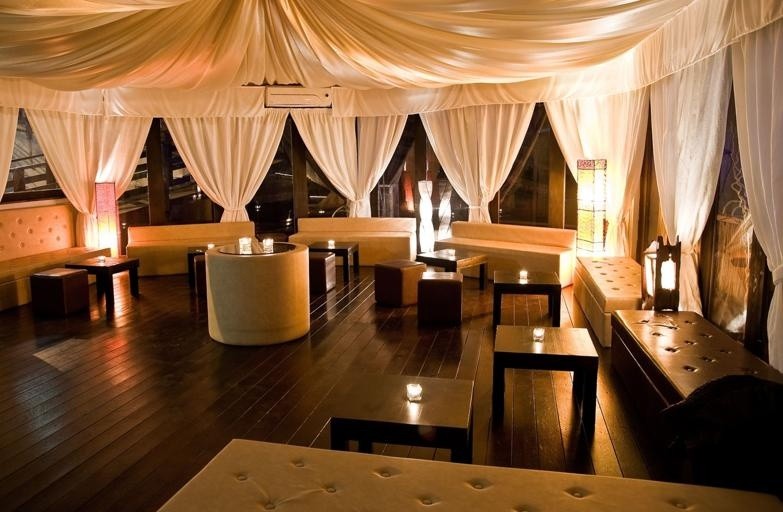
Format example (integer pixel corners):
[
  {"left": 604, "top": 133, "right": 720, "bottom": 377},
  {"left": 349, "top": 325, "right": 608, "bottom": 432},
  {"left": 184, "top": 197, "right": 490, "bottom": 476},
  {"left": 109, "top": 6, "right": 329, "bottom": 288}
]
[
  {"left": 127, "top": 222, "right": 258, "bottom": 278},
  {"left": 287, "top": 217, "right": 417, "bottom": 267},
  {"left": 431, "top": 220, "right": 576, "bottom": 287},
  {"left": 1, "top": 200, "right": 111, "bottom": 311}
]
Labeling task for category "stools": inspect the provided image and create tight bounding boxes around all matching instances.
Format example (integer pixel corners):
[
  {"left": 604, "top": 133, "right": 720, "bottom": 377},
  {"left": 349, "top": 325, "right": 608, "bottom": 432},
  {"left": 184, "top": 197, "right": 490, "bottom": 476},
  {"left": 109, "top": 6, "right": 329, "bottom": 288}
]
[
  {"left": 312, "top": 250, "right": 338, "bottom": 294},
  {"left": 372, "top": 259, "right": 426, "bottom": 307},
  {"left": 418, "top": 271, "right": 463, "bottom": 327},
  {"left": 30, "top": 268, "right": 90, "bottom": 324}
]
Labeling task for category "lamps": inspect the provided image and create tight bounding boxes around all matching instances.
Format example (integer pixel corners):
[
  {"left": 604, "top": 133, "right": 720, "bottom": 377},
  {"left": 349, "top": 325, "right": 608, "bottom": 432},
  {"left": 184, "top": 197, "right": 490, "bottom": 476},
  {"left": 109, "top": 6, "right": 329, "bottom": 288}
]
[
  {"left": 94, "top": 181, "right": 122, "bottom": 258},
  {"left": 577, "top": 159, "right": 607, "bottom": 258},
  {"left": 418, "top": 179, "right": 436, "bottom": 258},
  {"left": 642, "top": 235, "right": 682, "bottom": 312}
]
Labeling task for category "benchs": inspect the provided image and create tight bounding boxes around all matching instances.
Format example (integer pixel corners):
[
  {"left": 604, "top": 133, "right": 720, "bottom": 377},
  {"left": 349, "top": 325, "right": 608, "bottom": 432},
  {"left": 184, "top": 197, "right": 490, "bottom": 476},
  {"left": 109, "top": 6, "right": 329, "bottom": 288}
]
[
  {"left": 157, "top": 436, "right": 783, "bottom": 511},
  {"left": 574, "top": 252, "right": 646, "bottom": 347},
  {"left": 608, "top": 302, "right": 748, "bottom": 464}
]
